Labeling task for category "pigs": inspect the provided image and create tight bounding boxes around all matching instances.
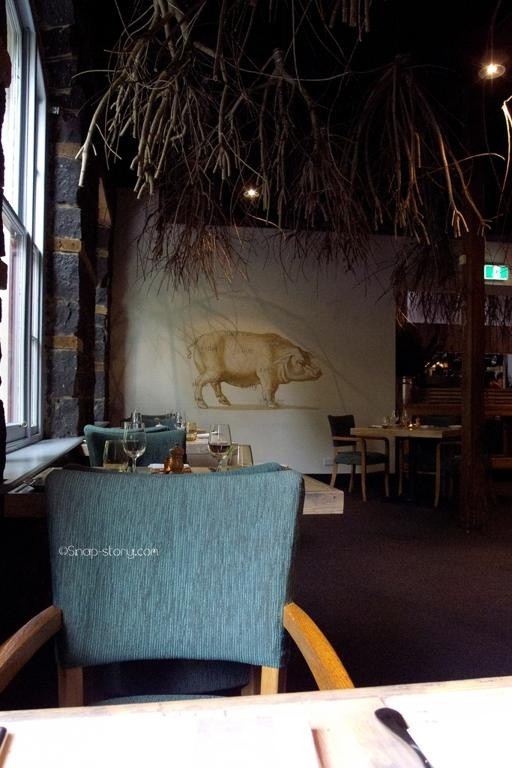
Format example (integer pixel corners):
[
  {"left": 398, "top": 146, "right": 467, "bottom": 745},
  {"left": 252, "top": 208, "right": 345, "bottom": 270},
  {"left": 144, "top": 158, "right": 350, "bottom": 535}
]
[{"left": 186, "top": 330, "right": 323, "bottom": 410}]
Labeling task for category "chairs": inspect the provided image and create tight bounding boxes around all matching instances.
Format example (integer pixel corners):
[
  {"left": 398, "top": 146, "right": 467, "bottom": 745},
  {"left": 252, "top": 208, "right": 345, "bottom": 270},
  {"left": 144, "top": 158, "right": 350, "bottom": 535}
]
[
  {"left": 325, "top": 408, "right": 393, "bottom": 502},
  {"left": 0, "top": 463, "right": 362, "bottom": 712},
  {"left": 80, "top": 412, "right": 241, "bottom": 468}
]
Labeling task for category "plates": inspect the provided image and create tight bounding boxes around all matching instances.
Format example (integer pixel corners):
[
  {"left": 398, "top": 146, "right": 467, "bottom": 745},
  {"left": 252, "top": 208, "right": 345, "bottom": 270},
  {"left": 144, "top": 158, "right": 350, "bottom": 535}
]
[{"left": 368, "top": 404, "right": 465, "bottom": 433}]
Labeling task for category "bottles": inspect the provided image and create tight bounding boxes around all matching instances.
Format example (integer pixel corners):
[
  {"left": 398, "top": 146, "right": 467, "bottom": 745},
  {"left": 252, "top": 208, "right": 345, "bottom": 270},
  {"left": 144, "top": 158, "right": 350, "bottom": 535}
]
[{"left": 402, "top": 375, "right": 418, "bottom": 404}]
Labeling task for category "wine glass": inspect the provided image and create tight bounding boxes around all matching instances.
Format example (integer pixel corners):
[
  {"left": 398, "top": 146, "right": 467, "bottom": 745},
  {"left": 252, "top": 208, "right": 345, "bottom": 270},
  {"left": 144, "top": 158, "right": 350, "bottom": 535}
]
[{"left": 101, "top": 407, "right": 254, "bottom": 473}]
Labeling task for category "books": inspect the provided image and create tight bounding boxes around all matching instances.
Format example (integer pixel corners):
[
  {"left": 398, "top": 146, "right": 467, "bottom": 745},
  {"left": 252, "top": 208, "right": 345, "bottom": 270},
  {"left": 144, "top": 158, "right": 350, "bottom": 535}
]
[{"left": 482, "top": 351, "right": 507, "bottom": 391}]
